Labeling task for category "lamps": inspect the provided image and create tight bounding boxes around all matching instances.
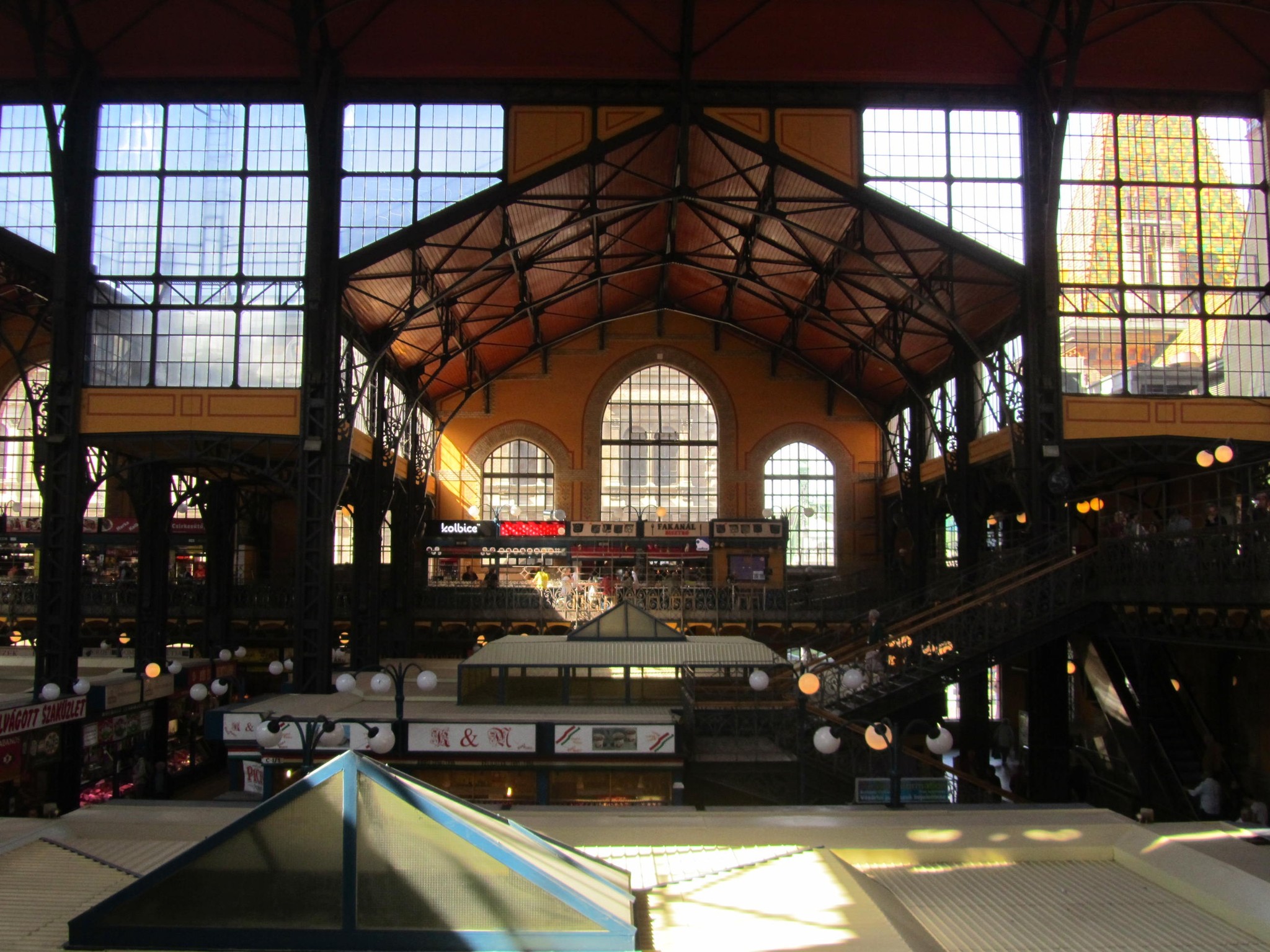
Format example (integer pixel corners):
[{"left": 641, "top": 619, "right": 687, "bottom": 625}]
[
  {"left": 9, "top": 631, "right": 22, "bottom": 642},
  {"left": 283, "top": 658, "right": 293, "bottom": 670},
  {"left": 42, "top": 682, "right": 60, "bottom": 700},
  {"left": 234, "top": 646, "right": 247, "bottom": 657},
  {"left": 474, "top": 630, "right": 529, "bottom": 649},
  {"left": 72, "top": 679, "right": 91, "bottom": 694},
  {"left": 256, "top": 669, "right": 438, "bottom": 754},
  {"left": 119, "top": 632, "right": 131, "bottom": 644},
  {"left": 219, "top": 649, "right": 232, "bottom": 661},
  {"left": 145, "top": 662, "right": 160, "bottom": 678},
  {"left": 211, "top": 679, "right": 228, "bottom": 696},
  {"left": 747, "top": 442, "right": 1234, "bottom": 757},
  {"left": 190, "top": 683, "right": 208, "bottom": 701},
  {"left": 469, "top": 505, "right": 667, "bottom": 522},
  {"left": 100, "top": 639, "right": 113, "bottom": 650},
  {"left": 268, "top": 661, "right": 283, "bottom": 675},
  {"left": 168, "top": 660, "right": 182, "bottom": 674}
]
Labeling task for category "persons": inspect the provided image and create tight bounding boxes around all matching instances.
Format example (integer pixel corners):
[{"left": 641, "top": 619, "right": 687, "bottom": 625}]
[
  {"left": 463, "top": 565, "right": 479, "bottom": 581},
  {"left": 865, "top": 606, "right": 889, "bottom": 687},
  {"left": 992, "top": 717, "right": 1012, "bottom": 767},
  {"left": 1104, "top": 491, "right": 1270, "bottom": 561},
  {"left": 483, "top": 566, "right": 498, "bottom": 581},
  {"left": 525, "top": 564, "right": 739, "bottom": 609},
  {"left": 1190, "top": 757, "right": 1223, "bottom": 820}
]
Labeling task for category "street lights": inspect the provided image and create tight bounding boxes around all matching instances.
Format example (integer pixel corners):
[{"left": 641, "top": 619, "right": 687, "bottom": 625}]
[
  {"left": 189, "top": 675, "right": 228, "bottom": 777},
  {"left": 1197, "top": 432, "right": 1248, "bottom": 583},
  {"left": 257, "top": 713, "right": 397, "bottom": 778},
  {"left": 809, "top": 716, "right": 956, "bottom": 808},
  {"left": 207, "top": 639, "right": 248, "bottom": 693},
  {"left": 747, "top": 659, "right": 864, "bottom": 805},
  {"left": 33, "top": 677, "right": 92, "bottom": 815},
  {"left": 987, "top": 489, "right": 1106, "bottom": 551},
  {"left": 336, "top": 660, "right": 439, "bottom": 766},
  {"left": 268, "top": 656, "right": 296, "bottom": 693},
  {"left": 129, "top": 652, "right": 183, "bottom": 747},
  {"left": 469, "top": 499, "right": 814, "bottom": 583}
]
